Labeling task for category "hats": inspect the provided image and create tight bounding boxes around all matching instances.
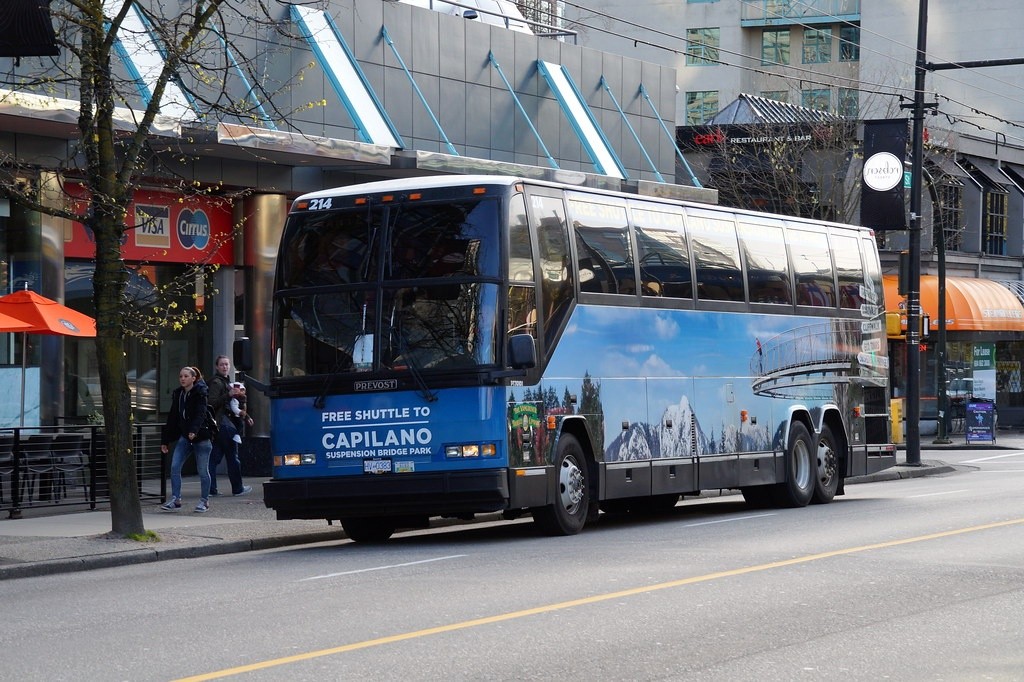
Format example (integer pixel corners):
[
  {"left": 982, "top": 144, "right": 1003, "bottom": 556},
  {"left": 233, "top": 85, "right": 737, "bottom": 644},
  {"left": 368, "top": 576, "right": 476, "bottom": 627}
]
[{"left": 229, "top": 382, "right": 246, "bottom": 390}]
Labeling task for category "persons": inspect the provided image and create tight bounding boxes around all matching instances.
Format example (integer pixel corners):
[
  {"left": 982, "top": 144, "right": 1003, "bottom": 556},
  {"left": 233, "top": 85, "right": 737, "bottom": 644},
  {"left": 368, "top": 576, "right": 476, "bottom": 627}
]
[
  {"left": 161, "top": 367, "right": 213, "bottom": 512},
  {"left": 209, "top": 356, "right": 254, "bottom": 497}
]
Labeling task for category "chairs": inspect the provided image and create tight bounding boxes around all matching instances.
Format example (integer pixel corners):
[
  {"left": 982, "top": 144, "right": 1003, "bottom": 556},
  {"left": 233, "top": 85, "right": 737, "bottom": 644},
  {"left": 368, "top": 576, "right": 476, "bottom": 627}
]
[
  {"left": 0, "top": 430, "right": 91, "bottom": 508},
  {"left": 947, "top": 394, "right": 999, "bottom": 434},
  {"left": 766, "top": 274, "right": 791, "bottom": 302}
]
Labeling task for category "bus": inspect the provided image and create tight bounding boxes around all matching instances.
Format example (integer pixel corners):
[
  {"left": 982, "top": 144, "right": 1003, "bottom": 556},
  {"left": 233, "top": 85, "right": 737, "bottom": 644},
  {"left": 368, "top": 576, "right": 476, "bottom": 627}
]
[{"left": 239, "top": 172, "right": 900, "bottom": 543}]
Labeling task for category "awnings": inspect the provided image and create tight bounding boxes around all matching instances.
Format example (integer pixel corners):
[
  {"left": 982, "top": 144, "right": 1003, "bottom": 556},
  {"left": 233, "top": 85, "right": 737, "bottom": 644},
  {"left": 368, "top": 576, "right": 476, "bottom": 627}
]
[{"left": 883, "top": 274, "right": 1024, "bottom": 342}]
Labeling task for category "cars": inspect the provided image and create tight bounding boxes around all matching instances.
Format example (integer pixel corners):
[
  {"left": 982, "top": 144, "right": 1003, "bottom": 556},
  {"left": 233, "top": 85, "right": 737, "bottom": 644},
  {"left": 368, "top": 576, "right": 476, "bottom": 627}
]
[{"left": 949, "top": 377, "right": 973, "bottom": 400}]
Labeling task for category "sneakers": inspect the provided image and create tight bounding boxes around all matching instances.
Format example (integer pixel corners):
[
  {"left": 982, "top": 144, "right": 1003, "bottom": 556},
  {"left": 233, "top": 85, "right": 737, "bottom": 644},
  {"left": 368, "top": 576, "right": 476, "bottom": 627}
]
[
  {"left": 233, "top": 485, "right": 252, "bottom": 496},
  {"left": 161, "top": 495, "right": 182, "bottom": 510},
  {"left": 195, "top": 497, "right": 209, "bottom": 512},
  {"left": 208, "top": 491, "right": 224, "bottom": 497}
]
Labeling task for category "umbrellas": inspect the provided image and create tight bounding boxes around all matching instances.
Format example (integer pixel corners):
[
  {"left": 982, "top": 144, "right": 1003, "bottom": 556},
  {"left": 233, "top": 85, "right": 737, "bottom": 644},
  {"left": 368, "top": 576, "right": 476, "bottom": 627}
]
[{"left": 0, "top": 282, "right": 96, "bottom": 427}]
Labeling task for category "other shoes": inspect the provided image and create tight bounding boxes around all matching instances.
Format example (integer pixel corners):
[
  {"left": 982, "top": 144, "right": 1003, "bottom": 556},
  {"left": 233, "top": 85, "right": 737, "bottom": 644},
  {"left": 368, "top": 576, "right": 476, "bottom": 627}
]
[{"left": 233, "top": 435, "right": 242, "bottom": 444}]
